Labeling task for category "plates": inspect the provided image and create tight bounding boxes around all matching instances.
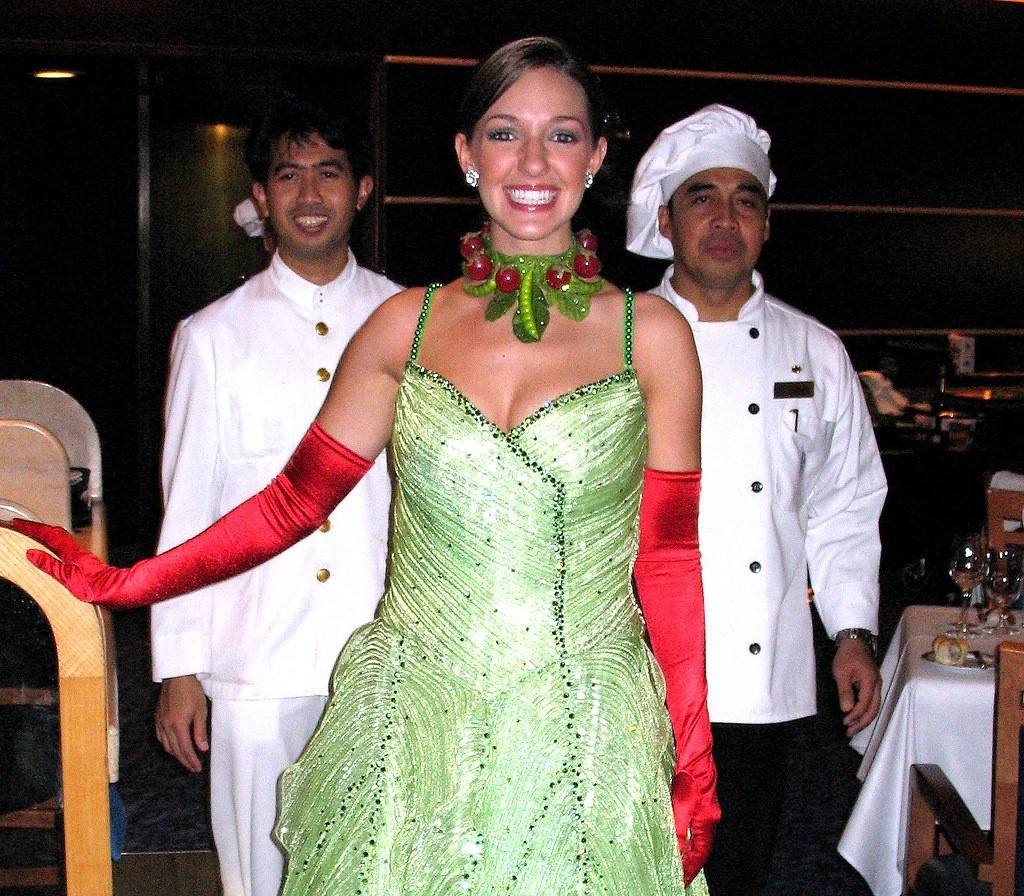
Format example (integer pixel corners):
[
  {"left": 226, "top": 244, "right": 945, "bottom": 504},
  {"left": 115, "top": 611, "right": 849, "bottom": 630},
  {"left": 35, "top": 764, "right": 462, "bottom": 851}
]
[{"left": 921, "top": 650, "right": 997, "bottom": 671}]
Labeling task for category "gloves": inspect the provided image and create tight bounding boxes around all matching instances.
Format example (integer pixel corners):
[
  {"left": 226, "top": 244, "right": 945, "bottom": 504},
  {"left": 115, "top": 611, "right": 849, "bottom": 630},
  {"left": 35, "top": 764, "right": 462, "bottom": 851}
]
[
  {"left": 634, "top": 464, "right": 722, "bottom": 888},
  {"left": 11, "top": 420, "right": 375, "bottom": 610}
]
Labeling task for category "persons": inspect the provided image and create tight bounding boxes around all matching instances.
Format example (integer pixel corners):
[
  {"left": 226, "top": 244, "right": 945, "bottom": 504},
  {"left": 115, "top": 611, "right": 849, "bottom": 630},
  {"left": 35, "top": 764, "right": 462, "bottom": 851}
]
[
  {"left": 11, "top": 37, "right": 724, "bottom": 896},
  {"left": 150, "top": 101, "right": 419, "bottom": 896},
  {"left": 627, "top": 103, "right": 888, "bottom": 896}
]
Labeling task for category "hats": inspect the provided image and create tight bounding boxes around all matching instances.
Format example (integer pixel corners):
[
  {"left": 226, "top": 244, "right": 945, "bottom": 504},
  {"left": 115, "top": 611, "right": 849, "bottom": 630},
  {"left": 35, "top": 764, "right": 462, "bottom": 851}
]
[{"left": 625, "top": 100, "right": 777, "bottom": 260}]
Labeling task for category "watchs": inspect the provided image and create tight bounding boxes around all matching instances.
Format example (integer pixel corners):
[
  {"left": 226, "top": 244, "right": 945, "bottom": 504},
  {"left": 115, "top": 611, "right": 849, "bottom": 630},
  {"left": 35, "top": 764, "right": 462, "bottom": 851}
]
[{"left": 834, "top": 627, "right": 879, "bottom": 659}]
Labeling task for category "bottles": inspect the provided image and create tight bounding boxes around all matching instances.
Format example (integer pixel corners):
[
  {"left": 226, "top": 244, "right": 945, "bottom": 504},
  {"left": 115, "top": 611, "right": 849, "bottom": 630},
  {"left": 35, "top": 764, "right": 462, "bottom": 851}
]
[{"left": 928, "top": 369, "right": 952, "bottom": 447}]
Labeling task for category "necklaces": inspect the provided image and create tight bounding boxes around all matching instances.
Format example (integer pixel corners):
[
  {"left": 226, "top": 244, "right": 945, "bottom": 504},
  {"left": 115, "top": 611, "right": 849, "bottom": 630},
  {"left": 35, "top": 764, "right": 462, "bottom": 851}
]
[{"left": 459, "top": 221, "right": 606, "bottom": 343}]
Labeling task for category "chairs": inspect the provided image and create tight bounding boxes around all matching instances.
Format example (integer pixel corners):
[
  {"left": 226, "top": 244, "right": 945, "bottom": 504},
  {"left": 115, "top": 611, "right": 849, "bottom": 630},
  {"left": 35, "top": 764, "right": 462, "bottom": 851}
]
[
  {"left": 854, "top": 367, "right": 972, "bottom": 449},
  {"left": 984, "top": 488, "right": 1024, "bottom": 568},
  {"left": 902, "top": 637, "right": 1024, "bottom": 896}
]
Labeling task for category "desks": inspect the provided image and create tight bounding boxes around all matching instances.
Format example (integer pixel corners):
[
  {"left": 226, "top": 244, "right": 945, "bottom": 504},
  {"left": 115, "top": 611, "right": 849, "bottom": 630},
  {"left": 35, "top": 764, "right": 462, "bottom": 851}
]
[{"left": 835, "top": 604, "right": 1024, "bottom": 896}]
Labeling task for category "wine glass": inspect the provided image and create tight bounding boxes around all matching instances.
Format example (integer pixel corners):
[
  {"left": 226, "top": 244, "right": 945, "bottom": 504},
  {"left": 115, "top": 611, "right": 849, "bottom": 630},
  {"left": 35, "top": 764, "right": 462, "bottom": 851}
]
[{"left": 945, "top": 539, "right": 1024, "bottom": 640}]
